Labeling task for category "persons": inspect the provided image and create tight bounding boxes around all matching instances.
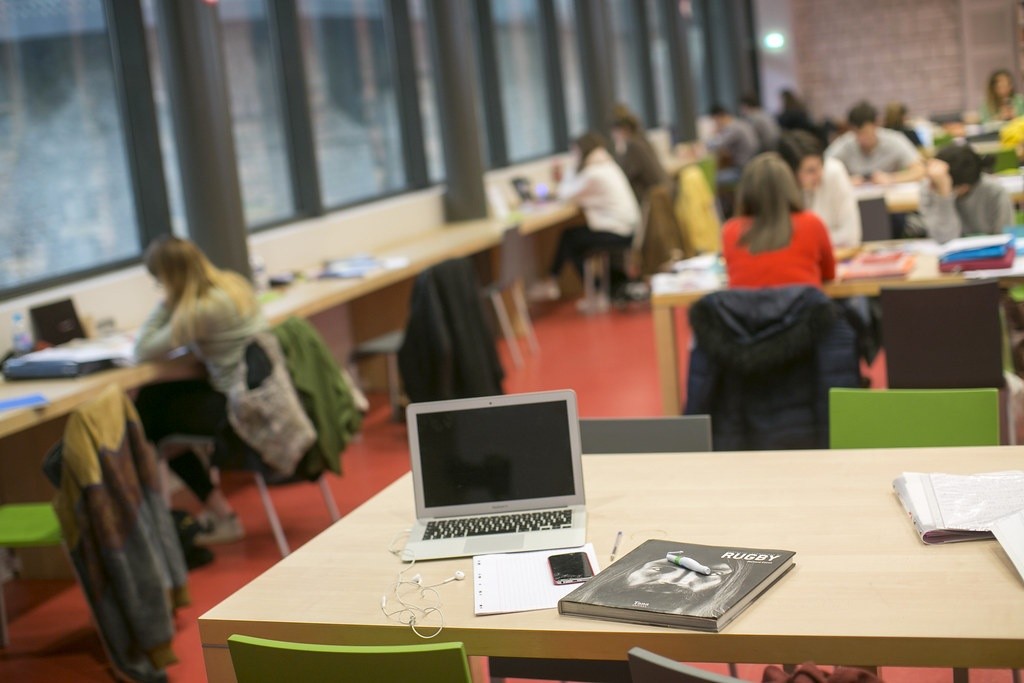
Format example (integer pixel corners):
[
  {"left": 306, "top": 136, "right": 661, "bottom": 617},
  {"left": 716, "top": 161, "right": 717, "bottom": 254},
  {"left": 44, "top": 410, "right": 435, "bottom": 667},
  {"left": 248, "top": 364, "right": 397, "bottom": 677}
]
[
  {"left": 981, "top": 73, "right": 1024, "bottom": 125},
  {"left": 529, "top": 133, "right": 640, "bottom": 312},
  {"left": 774, "top": 130, "right": 864, "bottom": 243},
  {"left": 825, "top": 101, "right": 923, "bottom": 186},
  {"left": 130, "top": 233, "right": 269, "bottom": 543},
  {"left": 707, "top": 88, "right": 811, "bottom": 190},
  {"left": 882, "top": 102, "right": 922, "bottom": 150},
  {"left": 918, "top": 145, "right": 1013, "bottom": 244},
  {"left": 721, "top": 154, "right": 838, "bottom": 292},
  {"left": 609, "top": 117, "right": 665, "bottom": 205}
]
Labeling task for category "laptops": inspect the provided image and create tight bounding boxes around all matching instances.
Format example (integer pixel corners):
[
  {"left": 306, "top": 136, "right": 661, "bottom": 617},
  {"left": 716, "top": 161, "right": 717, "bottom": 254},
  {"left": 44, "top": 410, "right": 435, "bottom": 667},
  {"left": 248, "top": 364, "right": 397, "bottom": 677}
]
[{"left": 401, "top": 389, "right": 586, "bottom": 562}]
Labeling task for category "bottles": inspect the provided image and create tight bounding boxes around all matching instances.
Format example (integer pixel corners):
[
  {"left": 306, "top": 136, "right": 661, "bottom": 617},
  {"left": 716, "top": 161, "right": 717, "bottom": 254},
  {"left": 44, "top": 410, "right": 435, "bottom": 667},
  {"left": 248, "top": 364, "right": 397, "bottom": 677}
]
[{"left": 12, "top": 311, "right": 32, "bottom": 353}]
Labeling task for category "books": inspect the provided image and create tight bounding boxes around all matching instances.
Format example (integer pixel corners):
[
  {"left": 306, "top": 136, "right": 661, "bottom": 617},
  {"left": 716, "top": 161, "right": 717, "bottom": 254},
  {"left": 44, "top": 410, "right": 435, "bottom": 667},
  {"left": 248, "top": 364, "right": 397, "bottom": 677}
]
[
  {"left": 831, "top": 233, "right": 1017, "bottom": 283},
  {"left": 558, "top": 538, "right": 796, "bottom": 633}
]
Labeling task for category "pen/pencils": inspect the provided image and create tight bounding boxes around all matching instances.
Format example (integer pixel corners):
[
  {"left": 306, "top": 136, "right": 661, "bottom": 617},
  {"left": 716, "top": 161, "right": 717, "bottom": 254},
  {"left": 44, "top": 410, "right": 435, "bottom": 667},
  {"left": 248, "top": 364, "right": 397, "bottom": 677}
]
[
  {"left": 609, "top": 531, "right": 622, "bottom": 562},
  {"left": 667, "top": 551, "right": 711, "bottom": 575}
]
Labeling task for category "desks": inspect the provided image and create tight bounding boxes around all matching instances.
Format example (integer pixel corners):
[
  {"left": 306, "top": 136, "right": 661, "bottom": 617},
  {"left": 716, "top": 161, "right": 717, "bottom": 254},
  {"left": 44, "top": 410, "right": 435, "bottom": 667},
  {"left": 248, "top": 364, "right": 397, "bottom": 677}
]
[
  {"left": 648, "top": 230, "right": 1024, "bottom": 420},
  {"left": 0, "top": 195, "right": 581, "bottom": 575},
  {"left": 854, "top": 172, "right": 1024, "bottom": 216},
  {"left": 198, "top": 439, "right": 1022, "bottom": 683}
]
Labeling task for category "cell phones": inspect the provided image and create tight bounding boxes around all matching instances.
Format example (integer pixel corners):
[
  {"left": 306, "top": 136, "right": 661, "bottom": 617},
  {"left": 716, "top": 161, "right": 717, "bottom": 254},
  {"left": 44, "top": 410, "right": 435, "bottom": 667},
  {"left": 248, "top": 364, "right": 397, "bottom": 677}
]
[{"left": 548, "top": 552, "right": 595, "bottom": 585}]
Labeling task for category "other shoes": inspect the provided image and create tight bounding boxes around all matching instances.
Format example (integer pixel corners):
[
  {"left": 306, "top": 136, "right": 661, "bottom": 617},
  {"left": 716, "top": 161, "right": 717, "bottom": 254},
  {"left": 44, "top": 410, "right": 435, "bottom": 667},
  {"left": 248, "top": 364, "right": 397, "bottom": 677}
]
[
  {"left": 523, "top": 283, "right": 560, "bottom": 304},
  {"left": 573, "top": 294, "right": 611, "bottom": 311},
  {"left": 194, "top": 509, "right": 245, "bottom": 545}
]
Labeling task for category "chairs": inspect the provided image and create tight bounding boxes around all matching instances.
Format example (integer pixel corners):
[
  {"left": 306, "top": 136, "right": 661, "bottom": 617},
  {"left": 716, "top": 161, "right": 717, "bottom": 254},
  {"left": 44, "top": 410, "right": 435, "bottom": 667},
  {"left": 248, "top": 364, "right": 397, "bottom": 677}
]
[
  {"left": 229, "top": 279, "right": 1023, "bottom": 683},
  {"left": 0, "top": 380, "right": 129, "bottom": 650}
]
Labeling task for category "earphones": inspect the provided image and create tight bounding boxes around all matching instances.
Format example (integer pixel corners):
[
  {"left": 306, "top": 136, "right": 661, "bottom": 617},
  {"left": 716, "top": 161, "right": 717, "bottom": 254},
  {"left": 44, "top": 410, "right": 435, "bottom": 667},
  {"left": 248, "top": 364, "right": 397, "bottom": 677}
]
[
  {"left": 444, "top": 571, "right": 465, "bottom": 583},
  {"left": 411, "top": 574, "right": 422, "bottom": 585}
]
